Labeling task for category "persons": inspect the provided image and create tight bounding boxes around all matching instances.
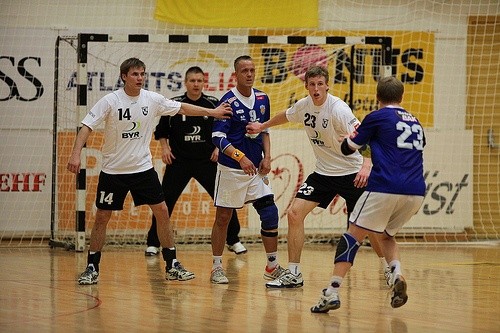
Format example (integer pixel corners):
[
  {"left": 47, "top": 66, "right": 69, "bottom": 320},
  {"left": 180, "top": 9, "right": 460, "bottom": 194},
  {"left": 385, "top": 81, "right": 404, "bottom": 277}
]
[
  {"left": 245, "top": 68, "right": 393, "bottom": 288},
  {"left": 209, "top": 55, "right": 285, "bottom": 284},
  {"left": 310, "top": 76, "right": 426, "bottom": 314},
  {"left": 65, "top": 59, "right": 234, "bottom": 285},
  {"left": 144, "top": 66, "right": 247, "bottom": 256}
]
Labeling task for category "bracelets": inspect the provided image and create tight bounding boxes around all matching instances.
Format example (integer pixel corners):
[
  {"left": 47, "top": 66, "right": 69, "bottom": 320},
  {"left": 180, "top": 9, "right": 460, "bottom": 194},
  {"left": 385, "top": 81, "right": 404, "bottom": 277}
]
[{"left": 231, "top": 149, "right": 245, "bottom": 162}]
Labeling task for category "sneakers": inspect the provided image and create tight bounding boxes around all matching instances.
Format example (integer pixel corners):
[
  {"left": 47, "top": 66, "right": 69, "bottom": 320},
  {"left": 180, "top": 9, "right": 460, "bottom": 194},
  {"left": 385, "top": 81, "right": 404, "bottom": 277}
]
[
  {"left": 166, "top": 259, "right": 195, "bottom": 281},
  {"left": 78, "top": 264, "right": 99, "bottom": 285},
  {"left": 263, "top": 264, "right": 286, "bottom": 280},
  {"left": 390, "top": 274, "right": 408, "bottom": 308},
  {"left": 311, "top": 289, "right": 340, "bottom": 313},
  {"left": 265, "top": 268, "right": 304, "bottom": 288},
  {"left": 225, "top": 241, "right": 247, "bottom": 254},
  {"left": 210, "top": 266, "right": 229, "bottom": 284},
  {"left": 384, "top": 267, "right": 392, "bottom": 285},
  {"left": 145, "top": 246, "right": 160, "bottom": 256}
]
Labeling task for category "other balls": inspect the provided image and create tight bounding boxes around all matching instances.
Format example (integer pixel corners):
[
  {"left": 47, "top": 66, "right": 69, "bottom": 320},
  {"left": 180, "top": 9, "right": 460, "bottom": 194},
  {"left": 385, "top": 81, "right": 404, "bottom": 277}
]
[{"left": 358, "top": 144, "right": 372, "bottom": 158}]
